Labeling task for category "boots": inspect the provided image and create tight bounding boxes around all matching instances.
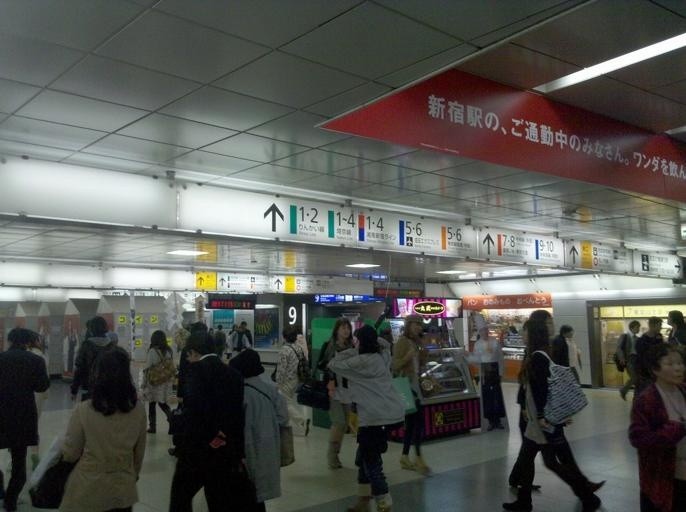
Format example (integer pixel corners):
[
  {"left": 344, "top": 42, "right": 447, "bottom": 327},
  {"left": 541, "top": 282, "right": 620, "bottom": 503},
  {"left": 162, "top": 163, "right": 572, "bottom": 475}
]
[
  {"left": 147, "top": 413, "right": 156, "bottom": 434},
  {"left": 166, "top": 409, "right": 176, "bottom": 434},
  {"left": 327, "top": 440, "right": 344, "bottom": 469},
  {"left": 346, "top": 483, "right": 373, "bottom": 512},
  {"left": 373, "top": 493, "right": 393, "bottom": 512}
]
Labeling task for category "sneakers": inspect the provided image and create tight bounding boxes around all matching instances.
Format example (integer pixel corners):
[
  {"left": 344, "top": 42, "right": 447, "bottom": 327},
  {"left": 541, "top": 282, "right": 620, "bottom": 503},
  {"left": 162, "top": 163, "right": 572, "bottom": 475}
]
[
  {"left": 502, "top": 500, "right": 532, "bottom": 512},
  {"left": 300, "top": 417, "right": 310, "bottom": 437},
  {"left": 399, "top": 455, "right": 416, "bottom": 471},
  {"left": 3, "top": 493, "right": 19, "bottom": 511},
  {"left": 618, "top": 388, "right": 628, "bottom": 402},
  {"left": 414, "top": 459, "right": 431, "bottom": 475}
]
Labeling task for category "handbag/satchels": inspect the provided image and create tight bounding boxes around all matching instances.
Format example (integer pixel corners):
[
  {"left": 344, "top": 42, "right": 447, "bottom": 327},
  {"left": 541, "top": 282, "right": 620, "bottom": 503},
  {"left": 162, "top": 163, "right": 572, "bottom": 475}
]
[
  {"left": 389, "top": 367, "right": 418, "bottom": 415},
  {"left": 28, "top": 454, "right": 77, "bottom": 510},
  {"left": 297, "top": 373, "right": 329, "bottom": 411},
  {"left": 317, "top": 341, "right": 331, "bottom": 371},
  {"left": 277, "top": 419, "right": 296, "bottom": 469},
  {"left": 271, "top": 366, "right": 277, "bottom": 383},
  {"left": 528, "top": 351, "right": 589, "bottom": 426},
  {"left": 142, "top": 357, "right": 174, "bottom": 386},
  {"left": 613, "top": 345, "right": 627, "bottom": 372}
]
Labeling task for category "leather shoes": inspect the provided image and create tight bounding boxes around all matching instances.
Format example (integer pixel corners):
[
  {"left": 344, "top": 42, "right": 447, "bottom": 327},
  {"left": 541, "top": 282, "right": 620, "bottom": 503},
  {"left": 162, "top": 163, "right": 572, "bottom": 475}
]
[
  {"left": 578, "top": 499, "right": 602, "bottom": 511},
  {"left": 487, "top": 421, "right": 506, "bottom": 430},
  {"left": 508, "top": 476, "right": 542, "bottom": 490},
  {"left": 575, "top": 479, "right": 607, "bottom": 497}
]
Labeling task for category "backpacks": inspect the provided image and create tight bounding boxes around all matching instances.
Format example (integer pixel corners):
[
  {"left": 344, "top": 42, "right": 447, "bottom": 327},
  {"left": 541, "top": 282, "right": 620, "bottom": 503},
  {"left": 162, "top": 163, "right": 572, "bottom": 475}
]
[{"left": 289, "top": 346, "right": 312, "bottom": 384}]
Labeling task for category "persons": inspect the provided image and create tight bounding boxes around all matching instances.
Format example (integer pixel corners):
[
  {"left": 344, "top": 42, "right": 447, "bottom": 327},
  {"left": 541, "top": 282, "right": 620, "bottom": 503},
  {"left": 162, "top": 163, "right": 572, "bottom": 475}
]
[
  {"left": 551, "top": 323, "right": 584, "bottom": 384},
  {"left": 506, "top": 307, "right": 607, "bottom": 494},
  {"left": 616, "top": 319, "right": 640, "bottom": 401},
  {"left": 502, "top": 319, "right": 603, "bottom": 511},
  {"left": 667, "top": 310, "right": 686, "bottom": 347},
  {"left": 626, "top": 345, "right": 686, "bottom": 512},
  {"left": 635, "top": 314, "right": 666, "bottom": 398},
  {"left": 1, "top": 314, "right": 434, "bottom": 512},
  {"left": 471, "top": 325, "right": 507, "bottom": 432}
]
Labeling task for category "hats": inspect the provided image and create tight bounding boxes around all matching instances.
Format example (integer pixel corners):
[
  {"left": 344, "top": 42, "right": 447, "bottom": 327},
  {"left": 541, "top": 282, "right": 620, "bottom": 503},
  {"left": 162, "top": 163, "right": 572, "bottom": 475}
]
[
  {"left": 473, "top": 313, "right": 488, "bottom": 333},
  {"left": 86, "top": 316, "right": 109, "bottom": 336},
  {"left": 229, "top": 348, "right": 265, "bottom": 378}
]
